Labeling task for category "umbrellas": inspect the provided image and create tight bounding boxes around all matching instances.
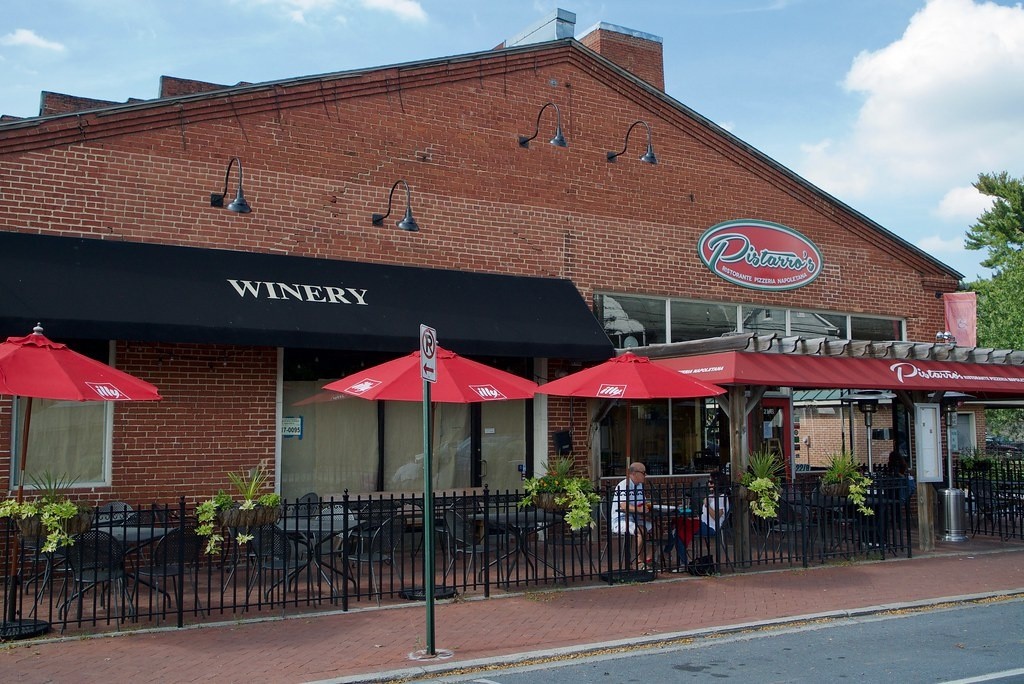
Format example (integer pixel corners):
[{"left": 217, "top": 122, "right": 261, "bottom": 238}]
[
  {"left": 3, "top": 322, "right": 161, "bottom": 640},
  {"left": 320, "top": 340, "right": 539, "bottom": 598},
  {"left": 532, "top": 344, "right": 727, "bottom": 584}
]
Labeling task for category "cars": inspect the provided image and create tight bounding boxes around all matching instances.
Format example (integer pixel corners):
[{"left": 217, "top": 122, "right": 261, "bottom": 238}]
[{"left": 986, "top": 435, "right": 1024, "bottom": 460}]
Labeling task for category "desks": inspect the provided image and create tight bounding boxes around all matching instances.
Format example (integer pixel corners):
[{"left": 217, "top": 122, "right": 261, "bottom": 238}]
[
  {"left": 469, "top": 512, "right": 567, "bottom": 593},
  {"left": 258, "top": 518, "right": 368, "bottom": 608},
  {"left": 69, "top": 526, "right": 178, "bottom": 610}
]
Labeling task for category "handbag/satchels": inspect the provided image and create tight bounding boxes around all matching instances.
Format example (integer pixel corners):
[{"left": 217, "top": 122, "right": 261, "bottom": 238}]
[{"left": 688, "top": 555, "right": 715, "bottom": 576}]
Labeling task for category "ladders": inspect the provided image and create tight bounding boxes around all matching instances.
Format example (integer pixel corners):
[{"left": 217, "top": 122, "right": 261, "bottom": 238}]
[{"left": 765, "top": 438, "right": 784, "bottom": 468}]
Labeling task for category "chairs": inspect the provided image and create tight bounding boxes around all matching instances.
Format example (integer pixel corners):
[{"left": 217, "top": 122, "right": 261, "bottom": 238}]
[
  {"left": 597, "top": 492, "right": 902, "bottom": 578},
  {"left": 222, "top": 491, "right": 319, "bottom": 593},
  {"left": 293, "top": 504, "right": 357, "bottom": 594},
  {"left": 60, "top": 530, "right": 136, "bottom": 636},
  {"left": 240, "top": 523, "right": 317, "bottom": 618},
  {"left": 346, "top": 499, "right": 398, "bottom": 568},
  {"left": 333, "top": 514, "right": 408, "bottom": 608},
  {"left": 17, "top": 502, "right": 144, "bottom": 617},
  {"left": 542, "top": 505, "right": 598, "bottom": 584},
  {"left": 442, "top": 497, "right": 505, "bottom": 593},
  {"left": 969, "top": 476, "right": 1022, "bottom": 540},
  {"left": 126, "top": 525, "right": 206, "bottom": 628}
]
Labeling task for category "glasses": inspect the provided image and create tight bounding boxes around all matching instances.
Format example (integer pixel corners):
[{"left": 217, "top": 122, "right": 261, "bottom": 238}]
[
  {"left": 708, "top": 480, "right": 715, "bottom": 484},
  {"left": 634, "top": 470, "right": 646, "bottom": 475}
]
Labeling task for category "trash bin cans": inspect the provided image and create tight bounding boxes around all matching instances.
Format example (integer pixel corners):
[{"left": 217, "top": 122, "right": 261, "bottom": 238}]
[{"left": 936, "top": 487, "right": 970, "bottom": 542}]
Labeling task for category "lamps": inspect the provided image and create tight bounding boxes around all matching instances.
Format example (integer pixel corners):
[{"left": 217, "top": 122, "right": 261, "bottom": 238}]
[
  {"left": 372, "top": 179, "right": 420, "bottom": 232},
  {"left": 518, "top": 102, "right": 568, "bottom": 149},
  {"left": 606, "top": 119, "right": 658, "bottom": 165},
  {"left": 210, "top": 157, "right": 252, "bottom": 214}
]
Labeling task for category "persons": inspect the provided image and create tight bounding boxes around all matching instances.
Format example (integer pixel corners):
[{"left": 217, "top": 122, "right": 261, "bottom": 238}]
[
  {"left": 657, "top": 471, "right": 730, "bottom": 574},
  {"left": 610, "top": 462, "right": 654, "bottom": 571}
]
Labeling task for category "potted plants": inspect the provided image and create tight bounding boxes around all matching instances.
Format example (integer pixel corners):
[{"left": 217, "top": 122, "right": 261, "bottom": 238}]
[
  {"left": 817, "top": 447, "right": 876, "bottom": 516},
  {"left": 193, "top": 461, "right": 284, "bottom": 557},
  {"left": 516, "top": 447, "right": 603, "bottom": 531},
  {"left": 734, "top": 449, "right": 792, "bottom": 520},
  {"left": 0, "top": 467, "right": 95, "bottom": 553}
]
